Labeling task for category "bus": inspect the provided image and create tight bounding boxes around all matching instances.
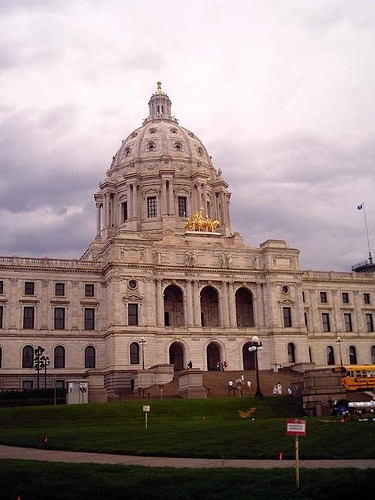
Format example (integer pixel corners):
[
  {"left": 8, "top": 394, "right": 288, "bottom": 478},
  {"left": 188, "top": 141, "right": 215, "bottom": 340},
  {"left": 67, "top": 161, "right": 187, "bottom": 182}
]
[{"left": 335, "top": 365, "right": 375, "bottom": 392}]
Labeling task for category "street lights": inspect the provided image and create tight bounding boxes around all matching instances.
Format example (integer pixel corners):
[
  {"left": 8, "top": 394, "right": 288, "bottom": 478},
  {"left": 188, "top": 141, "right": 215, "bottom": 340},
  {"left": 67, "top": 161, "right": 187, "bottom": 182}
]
[
  {"left": 138, "top": 337, "right": 147, "bottom": 370},
  {"left": 248, "top": 336, "right": 264, "bottom": 400},
  {"left": 335, "top": 337, "right": 343, "bottom": 366}
]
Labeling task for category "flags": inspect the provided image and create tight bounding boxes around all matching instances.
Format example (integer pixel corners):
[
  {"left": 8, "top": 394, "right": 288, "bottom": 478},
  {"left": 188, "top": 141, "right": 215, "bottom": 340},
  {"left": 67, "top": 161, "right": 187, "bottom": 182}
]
[{"left": 357, "top": 204, "right": 362, "bottom": 210}]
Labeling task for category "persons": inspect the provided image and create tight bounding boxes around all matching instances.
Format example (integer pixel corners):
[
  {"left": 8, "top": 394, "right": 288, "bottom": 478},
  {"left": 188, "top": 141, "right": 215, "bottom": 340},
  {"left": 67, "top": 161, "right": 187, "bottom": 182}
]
[
  {"left": 276, "top": 381, "right": 283, "bottom": 396},
  {"left": 331, "top": 399, "right": 375, "bottom": 423},
  {"left": 252, "top": 255, "right": 260, "bottom": 270},
  {"left": 183, "top": 248, "right": 190, "bottom": 266},
  {"left": 273, "top": 363, "right": 279, "bottom": 377},
  {"left": 215, "top": 359, "right": 229, "bottom": 371},
  {"left": 190, "top": 249, "right": 198, "bottom": 267},
  {"left": 154, "top": 247, "right": 162, "bottom": 265},
  {"left": 287, "top": 386, "right": 293, "bottom": 396},
  {"left": 188, "top": 361, "right": 192, "bottom": 369},
  {"left": 219, "top": 251, "right": 227, "bottom": 269},
  {"left": 226, "top": 373, "right": 253, "bottom": 393},
  {"left": 273, "top": 385, "right": 278, "bottom": 396},
  {"left": 227, "top": 251, "right": 233, "bottom": 269}
]
